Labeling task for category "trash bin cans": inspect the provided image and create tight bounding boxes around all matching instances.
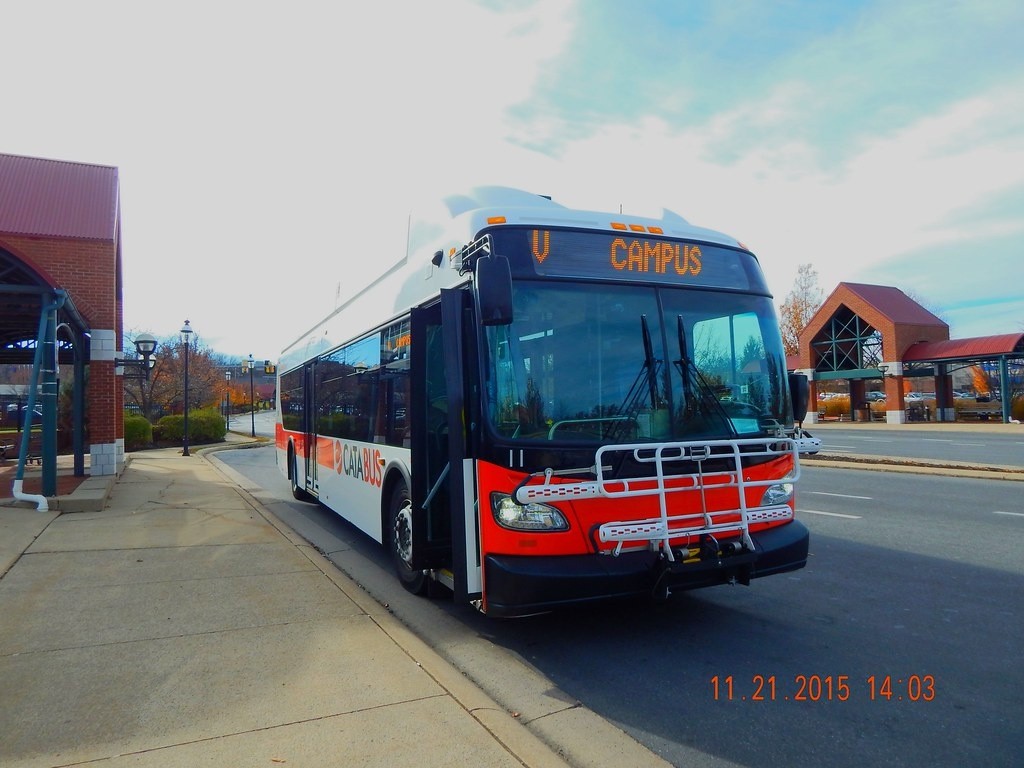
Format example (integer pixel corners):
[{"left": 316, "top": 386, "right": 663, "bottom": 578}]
[{"left": 856, "top": 401, "right": 871, "bottom": 422}]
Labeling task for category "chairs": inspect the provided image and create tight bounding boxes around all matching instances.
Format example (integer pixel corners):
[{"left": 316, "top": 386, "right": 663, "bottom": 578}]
[
  {"left": 0, "top": 438, "right": 17, "bottom": 465},
  {"left": 909, "top": 400, "right": 926, "bottom": 421}
]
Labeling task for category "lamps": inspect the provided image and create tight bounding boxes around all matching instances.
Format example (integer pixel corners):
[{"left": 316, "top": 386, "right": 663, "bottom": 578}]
[
  {"left": 115, "top": 353, "right": 156, "bottom": 370},
  {"left": 877, "top": 366, "right": 893, "bottom": 379},
  {"left": 115, "top": 333, "right": 157, "bottom": 380}
]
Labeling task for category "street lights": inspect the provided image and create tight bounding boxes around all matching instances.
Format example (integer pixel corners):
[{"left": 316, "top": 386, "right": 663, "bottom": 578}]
[
  {"left": 246, "top": 354, "right": 257, "bottom": 437},
  {"left": 180, "top": 319, "right": 194, "bottom": 456},
  {"left": 243, "top": 392, "right": 246, "bottom": 415},
  {"left": 256, "top": 392, "right": 259, "bottom": 413},
  {"left": 225, "top": 368, "right": 233, "bottom": 431}
]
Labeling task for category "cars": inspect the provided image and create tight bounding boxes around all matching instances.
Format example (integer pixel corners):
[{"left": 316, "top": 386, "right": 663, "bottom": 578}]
[{"left": 817, "top": 386, "right": 1024, "bottom": 423}]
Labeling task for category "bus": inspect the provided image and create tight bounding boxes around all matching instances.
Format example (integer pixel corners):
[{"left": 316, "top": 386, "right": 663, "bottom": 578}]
[{"left": 277, "top": 183, "right": 812, "bottom": 620}]
